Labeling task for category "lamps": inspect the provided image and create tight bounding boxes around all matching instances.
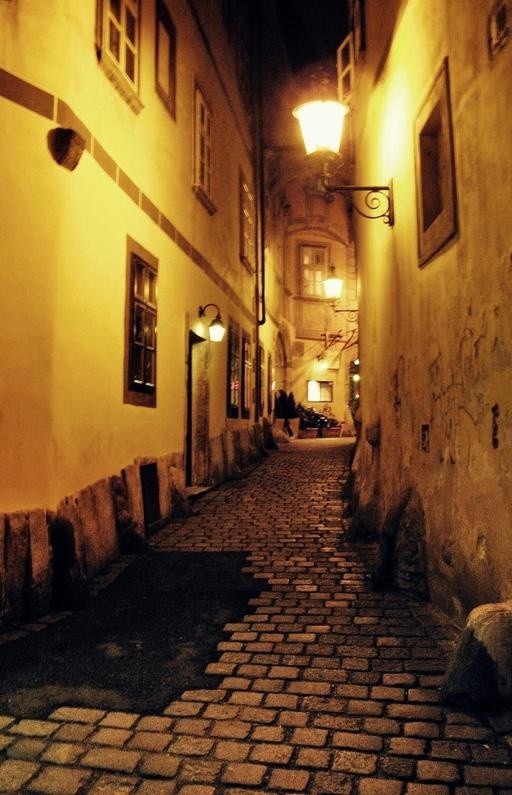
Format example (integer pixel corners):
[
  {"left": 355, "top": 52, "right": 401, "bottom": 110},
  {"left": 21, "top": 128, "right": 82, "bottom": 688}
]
[
  {"left": 292, "top": 100, "right": 394, "bottom": 228},
  {"left": 198, "top": 304, "right": 226, "bottom": 342}
]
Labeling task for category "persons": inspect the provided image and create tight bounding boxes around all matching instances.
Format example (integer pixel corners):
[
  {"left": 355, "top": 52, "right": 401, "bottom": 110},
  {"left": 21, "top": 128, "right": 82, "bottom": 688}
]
[{"left": 276, "top": 389, "right": 296, "bottom": 440}]
